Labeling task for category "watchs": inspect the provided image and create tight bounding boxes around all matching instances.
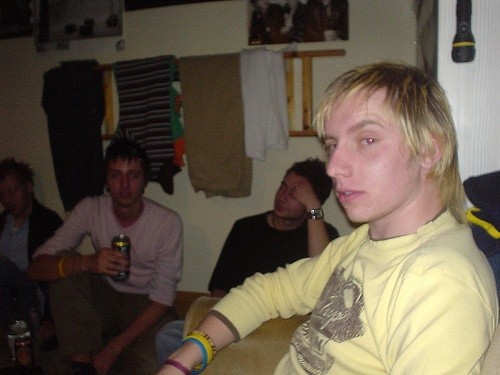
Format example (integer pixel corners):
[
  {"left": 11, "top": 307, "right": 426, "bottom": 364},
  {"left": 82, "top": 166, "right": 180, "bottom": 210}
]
[{"left": 304, "top": 208, "right": 323, "bottom": 220}]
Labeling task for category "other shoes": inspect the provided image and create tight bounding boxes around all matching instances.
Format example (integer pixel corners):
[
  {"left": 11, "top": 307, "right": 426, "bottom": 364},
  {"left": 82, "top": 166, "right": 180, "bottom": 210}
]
[{"left": 38, "top": 317, "right": 56, "bottom": 348}]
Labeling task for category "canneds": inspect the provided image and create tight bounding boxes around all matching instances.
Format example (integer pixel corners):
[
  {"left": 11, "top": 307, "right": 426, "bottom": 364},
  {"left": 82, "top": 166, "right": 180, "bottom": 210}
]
[
  {"left": 8, "top": 321, "right": 33, "bottom": 370},
  {"left": 110, "top": 235, "right": 131, "bottom": 281}
]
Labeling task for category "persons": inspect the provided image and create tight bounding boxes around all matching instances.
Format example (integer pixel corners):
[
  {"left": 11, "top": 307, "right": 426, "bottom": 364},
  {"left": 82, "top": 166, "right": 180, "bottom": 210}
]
[
  {"left": 26, "top": 136, "right": 185, "bottom": 375},
  {"left": 157, "top": 62, "right": 500, "bottom": 375},
  {"left": 208, "top": 156, "right": 340, "bottom": 297},
  {"left": 0, "top": 157, "right": 64, "bottom": 353}
]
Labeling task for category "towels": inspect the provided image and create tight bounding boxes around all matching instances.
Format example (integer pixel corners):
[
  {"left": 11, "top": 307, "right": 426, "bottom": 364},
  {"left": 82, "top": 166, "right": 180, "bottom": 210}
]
[
  {"left": 40, "top": 59, "right": 106, "bottom": 213},
  {"left": 177, "top": 53, "right": 252, "bottom": 198},
  {"left": 239, "top": 49, "right": 290, "bottom": 160},
  {"left": 115, "top": 55, "right": 177, "bottom": 182}
]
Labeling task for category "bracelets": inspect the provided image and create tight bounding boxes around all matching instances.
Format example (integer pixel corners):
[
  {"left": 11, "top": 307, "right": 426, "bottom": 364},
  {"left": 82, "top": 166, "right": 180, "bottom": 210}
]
[
  {"left": 184, "top": 330, "right": 216, "bottom": 375},
  {"left": 163, "top": 359, "right": 192, "bottom": 375},
  {"left": 57, "top": 256, "right": 69, "bottom": 280}
]
